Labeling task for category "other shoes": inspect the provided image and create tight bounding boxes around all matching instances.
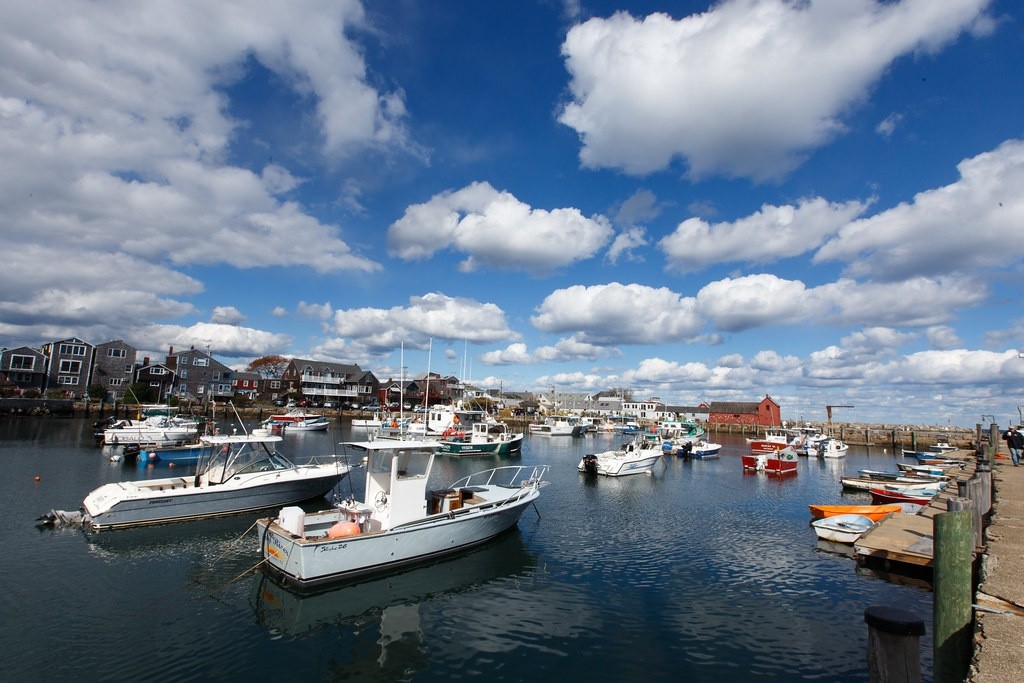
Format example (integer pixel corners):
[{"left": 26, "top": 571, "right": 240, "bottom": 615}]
[{"left": 1014, "top": 464, "right": 1019, "bottom": 467}]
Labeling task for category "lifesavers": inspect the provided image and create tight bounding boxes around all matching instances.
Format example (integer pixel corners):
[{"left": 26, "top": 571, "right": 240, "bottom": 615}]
[{"left": 453, "top": 414, "right": 460, "bottom": 425}]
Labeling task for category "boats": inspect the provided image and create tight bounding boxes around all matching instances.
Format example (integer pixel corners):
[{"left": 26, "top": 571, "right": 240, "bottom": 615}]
[
  {"left": 255, "top": 337, "right": 554, "bottom": 599},
  {"left": 748, "top": 405, "right": 967, "bottom": 496},
  {"left": 807, "top": 503, "right": 903, "bottom": 523},
  {"left": 741, "top": 445, "right": 800, "bottom": 475},
  {"left": 91, "top": 335, "right": 526, "bottom": 469},
  {"left": 576, "top": 434, "right": 666, "bottom": 478},
  {"left": 33, "top": 398, "right": 356, "bottom": 534},
  {"left": 811, "top": 514, "right": 875, "bottom": 543},
  {"left": 869, "top": 488, "right": 934, "bottom": 506},
  {"left": 884, "top": 502, "right": 924, "bottom": 516},
  {"left": 528, "top": 381, "right": 722, "bottom": 463}
]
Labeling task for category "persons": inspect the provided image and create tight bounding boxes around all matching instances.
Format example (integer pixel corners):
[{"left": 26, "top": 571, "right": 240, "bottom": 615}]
[{"left": 1001, "top": 425, "right": 1024, "bottom": 467}]
